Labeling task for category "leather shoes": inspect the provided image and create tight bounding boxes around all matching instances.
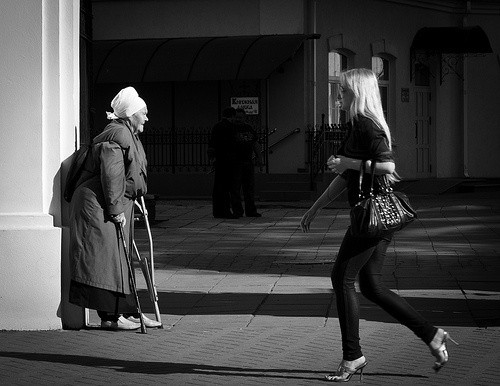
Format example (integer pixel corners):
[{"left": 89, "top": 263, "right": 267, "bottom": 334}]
[
  {"left": 100, "top": 313, "right": 142, "bottom": 330},
  {"left": 126, "top": 314, "right": 163, "bottom": 328}
]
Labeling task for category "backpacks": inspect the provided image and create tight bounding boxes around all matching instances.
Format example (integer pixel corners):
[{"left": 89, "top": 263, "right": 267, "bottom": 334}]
[{"left": 64, "top": 139, "right": 126, "bottom": 203}]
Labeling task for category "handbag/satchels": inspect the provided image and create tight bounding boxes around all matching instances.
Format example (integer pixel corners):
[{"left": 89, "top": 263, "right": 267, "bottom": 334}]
[{"left": 352, "top": 158, "right": 417, "bottom": 240}]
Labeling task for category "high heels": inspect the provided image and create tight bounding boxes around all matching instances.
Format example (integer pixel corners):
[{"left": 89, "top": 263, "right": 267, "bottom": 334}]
[
  {"left": 324, "top": 361, "right": 368, "bottom": 382},
  {"left": 432, "top": 331, "right": 458, "bottom": 370}
]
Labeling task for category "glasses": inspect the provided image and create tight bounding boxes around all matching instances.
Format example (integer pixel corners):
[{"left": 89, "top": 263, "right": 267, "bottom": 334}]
[{"left": 338, "top": 86, "right": 349, "bottom": 94}]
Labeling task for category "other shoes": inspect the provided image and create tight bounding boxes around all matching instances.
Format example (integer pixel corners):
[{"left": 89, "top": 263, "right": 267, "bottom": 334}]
[{"left": 213, "top": 210, "right": 261, "bottom": 219}]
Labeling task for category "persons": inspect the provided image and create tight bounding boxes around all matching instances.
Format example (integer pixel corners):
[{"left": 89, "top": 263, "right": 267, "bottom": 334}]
[
  {"left": 206, "top": 107, "right": 263, "bottom": 219},
  {"left": 68, "top": 86, "right": 163, "bottom": 334},
  {"left": 300, "top": 68, "right": 459, "bottom": 383}
]
